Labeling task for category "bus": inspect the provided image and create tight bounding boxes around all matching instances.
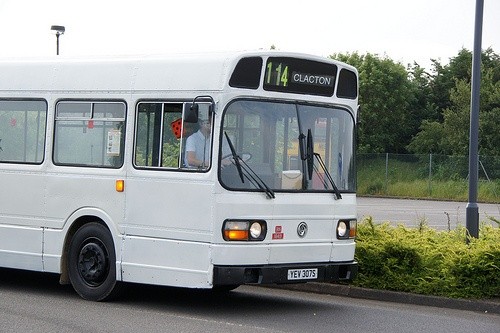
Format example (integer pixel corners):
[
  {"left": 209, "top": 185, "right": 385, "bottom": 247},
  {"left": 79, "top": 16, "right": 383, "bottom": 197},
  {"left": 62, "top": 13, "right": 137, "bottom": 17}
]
[{"left": 0, "top": 47, "right": 362, "bottom": 302}]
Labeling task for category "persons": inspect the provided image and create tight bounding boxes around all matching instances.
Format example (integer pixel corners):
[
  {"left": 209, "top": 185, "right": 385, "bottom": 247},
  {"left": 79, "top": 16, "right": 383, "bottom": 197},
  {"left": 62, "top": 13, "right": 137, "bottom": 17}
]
[{"left": 185, "top": 105, "right": 234, "bottom": 170}]
